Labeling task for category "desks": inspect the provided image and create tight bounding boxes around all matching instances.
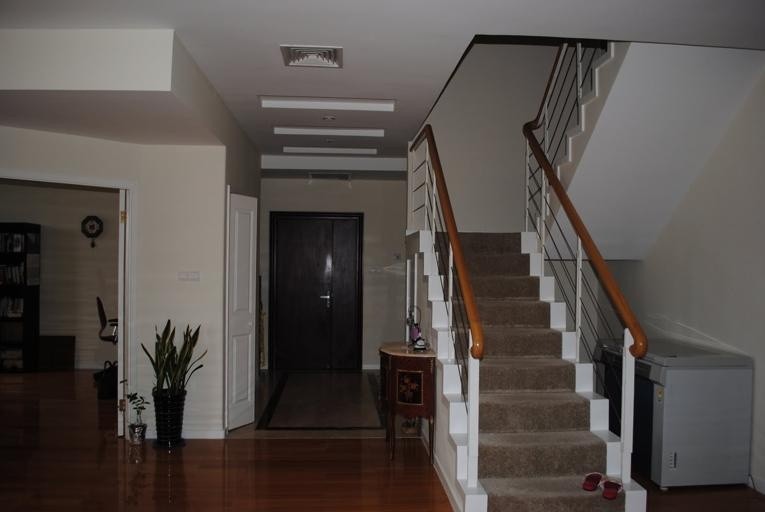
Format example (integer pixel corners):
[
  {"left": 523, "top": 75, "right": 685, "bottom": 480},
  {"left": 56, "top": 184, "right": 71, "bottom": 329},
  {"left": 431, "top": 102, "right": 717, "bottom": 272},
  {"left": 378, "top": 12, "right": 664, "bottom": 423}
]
[{"left": 378, "top": 341, "right": 438, "bottom": 465}]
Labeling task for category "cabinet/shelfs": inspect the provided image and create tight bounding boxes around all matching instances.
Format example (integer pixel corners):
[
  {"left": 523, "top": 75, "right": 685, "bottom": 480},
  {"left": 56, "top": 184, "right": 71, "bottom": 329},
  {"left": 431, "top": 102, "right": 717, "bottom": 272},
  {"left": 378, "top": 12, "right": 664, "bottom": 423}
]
[{"left": 0, "top": 222, "right": 42, "bottom": 374}]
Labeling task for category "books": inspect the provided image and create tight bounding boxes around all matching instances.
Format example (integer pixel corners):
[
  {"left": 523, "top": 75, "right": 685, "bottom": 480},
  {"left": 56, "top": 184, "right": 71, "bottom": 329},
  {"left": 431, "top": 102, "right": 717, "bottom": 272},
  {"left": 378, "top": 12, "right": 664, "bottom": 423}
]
[{"left": 0, "top": 232, "right": 24, "bottom": 318}]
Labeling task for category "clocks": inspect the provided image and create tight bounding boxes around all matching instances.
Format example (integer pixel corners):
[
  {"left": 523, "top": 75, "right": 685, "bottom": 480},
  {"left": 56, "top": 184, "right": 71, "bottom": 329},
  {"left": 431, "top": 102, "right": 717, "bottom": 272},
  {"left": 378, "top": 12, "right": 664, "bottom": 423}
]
[{"left": 81, "top": 216, "right": 103, "bottom": 248}]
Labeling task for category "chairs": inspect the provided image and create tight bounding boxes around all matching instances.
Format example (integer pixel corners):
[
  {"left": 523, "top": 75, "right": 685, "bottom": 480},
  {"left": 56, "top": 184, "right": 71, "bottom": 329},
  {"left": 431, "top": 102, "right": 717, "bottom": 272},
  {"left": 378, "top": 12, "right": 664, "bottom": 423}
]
[{"left": 95, "top": 296, "right": 119, "bottom": 361}]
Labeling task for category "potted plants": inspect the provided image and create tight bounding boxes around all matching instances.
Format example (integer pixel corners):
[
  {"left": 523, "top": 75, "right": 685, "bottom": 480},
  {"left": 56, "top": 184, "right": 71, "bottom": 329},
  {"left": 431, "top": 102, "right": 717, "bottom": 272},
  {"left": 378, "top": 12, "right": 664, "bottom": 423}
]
[
  {"left": 123, "top": 445, "right": 147, "bottom": 506},
  {"left": 139, "top": 318, "right": 207, "bottom": 450},
  {"left": 152, "top": 449, "right": 189, "bottom": 512},
  {"left": 126, "top": 393, "right": 151, "bottom": 445}
]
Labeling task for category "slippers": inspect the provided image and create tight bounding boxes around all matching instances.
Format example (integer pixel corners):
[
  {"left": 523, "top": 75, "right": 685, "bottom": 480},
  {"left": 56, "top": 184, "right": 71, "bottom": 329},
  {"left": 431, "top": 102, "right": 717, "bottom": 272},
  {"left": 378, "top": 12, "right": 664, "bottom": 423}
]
[{"left": 582, "top": 472, "right": 623, "bottom": 500}]
